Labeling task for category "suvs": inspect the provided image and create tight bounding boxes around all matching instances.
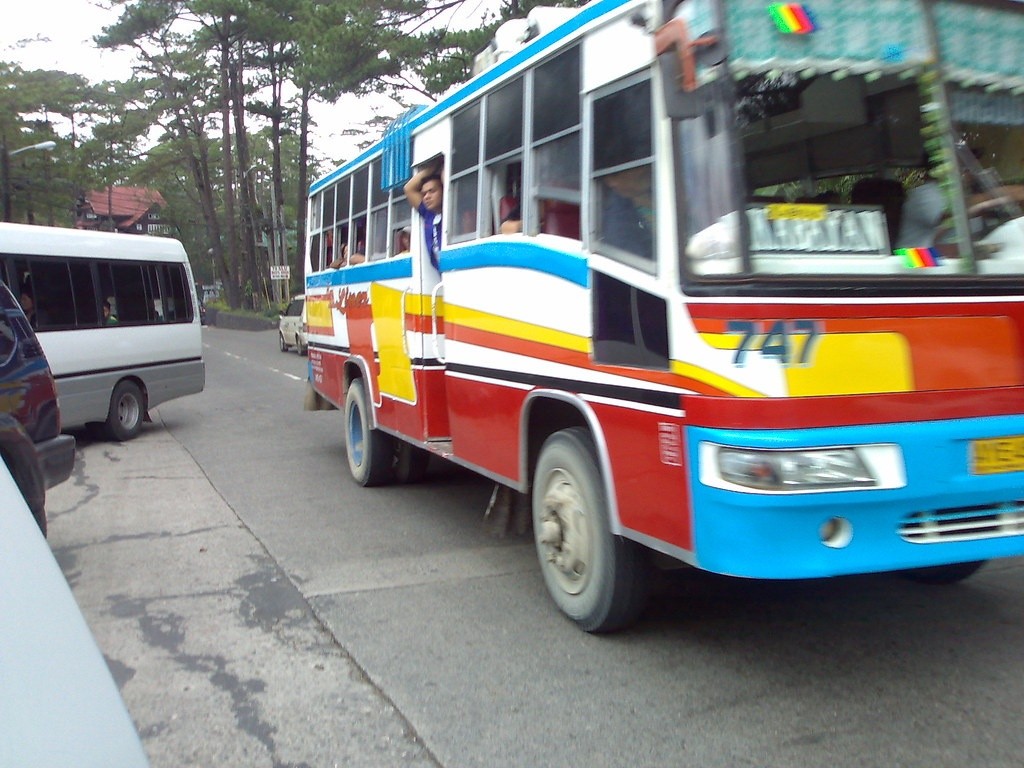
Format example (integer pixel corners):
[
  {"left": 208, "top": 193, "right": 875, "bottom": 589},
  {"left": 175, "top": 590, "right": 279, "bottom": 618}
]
[{"left": 0, "top": 282, "right": 76, "bottom": 538}]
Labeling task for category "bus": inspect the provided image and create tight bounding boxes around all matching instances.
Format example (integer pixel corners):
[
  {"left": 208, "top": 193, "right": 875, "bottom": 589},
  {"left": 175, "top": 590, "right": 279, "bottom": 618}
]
[
  {"left": 302, "top": 1, "right": 1023, "bottom": 635},
  {"left": 1, "top": 220, "right": 208, "bottom": 441}
]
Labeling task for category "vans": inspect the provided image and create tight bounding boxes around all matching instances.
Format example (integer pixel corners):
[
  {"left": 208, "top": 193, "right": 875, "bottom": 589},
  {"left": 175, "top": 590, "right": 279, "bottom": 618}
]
[{"left": 278, "top": 293, "right": 307, "bottom": 356}]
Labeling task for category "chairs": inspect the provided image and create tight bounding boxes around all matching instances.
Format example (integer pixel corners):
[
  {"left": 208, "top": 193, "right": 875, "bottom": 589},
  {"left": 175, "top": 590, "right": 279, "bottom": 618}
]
[{"left": 853, "top": 178, "right": 906, "bottom": 247}]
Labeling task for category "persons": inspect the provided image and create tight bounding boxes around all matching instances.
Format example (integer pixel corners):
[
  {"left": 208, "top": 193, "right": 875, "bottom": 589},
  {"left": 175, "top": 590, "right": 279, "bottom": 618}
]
[
  {"left": 103, "top": 300, "right": 119, "bottom": 325},
  {"left": 330, "top": 225, "right": 411, "bottom": 268},
  {"left": 195, "top": 279, "right": 204, "bottom": 305},
  {"left": 899, "top": 146, "right": 1024, "bottom": 249},
  {"left": 20, "top": 289, "right": 36, "bottom": 328},
  {"left": 603, "top": 155, "right": 687, "bottom": 266},
  {"left": 501, "top": 198, "right": 546, "bottom": 234},
  {"left": 403, "top": 156, "right": 444, "bottom": 281}
]
